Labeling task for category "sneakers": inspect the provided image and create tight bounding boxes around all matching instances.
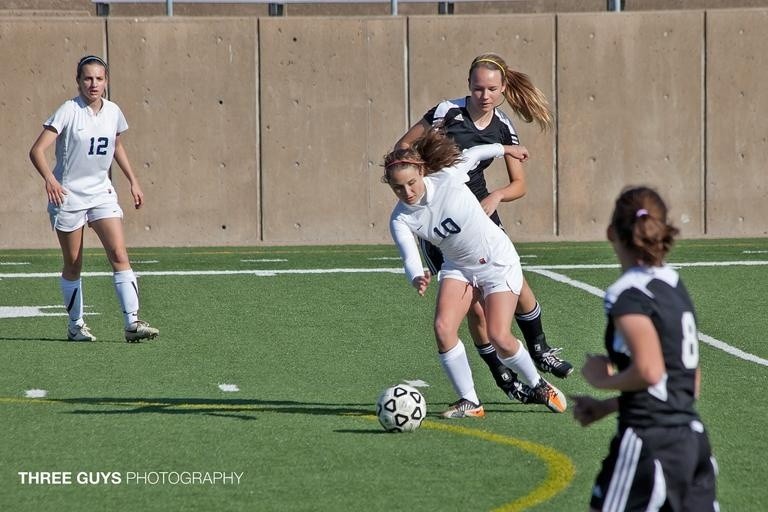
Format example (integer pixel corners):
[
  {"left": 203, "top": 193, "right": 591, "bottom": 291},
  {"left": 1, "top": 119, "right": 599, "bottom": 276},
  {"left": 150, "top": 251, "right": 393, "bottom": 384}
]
[
  {"left": 440, "top": 398, "right": 485, "bottom": 419},
  {"left": 125, "top": 320, "right": 159, "bottom": 343},
  {"left": 508, "top": 381, "right": 544, "bottom": 403},
  {"left": 534, "top": 348, "right": 574, "bottom": 379},
  {"left": 68, "top": 324, "right": 96, "bottom": 341},
  {"left": 531, "top": 377, "right": 567, "bottom": 413}
]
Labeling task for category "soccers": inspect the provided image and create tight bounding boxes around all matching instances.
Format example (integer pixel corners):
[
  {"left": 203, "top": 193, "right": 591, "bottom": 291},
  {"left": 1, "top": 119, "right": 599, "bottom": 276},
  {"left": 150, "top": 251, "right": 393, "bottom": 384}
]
[{"left": 376, "top": 384, "right": 427, "bottom": 433}]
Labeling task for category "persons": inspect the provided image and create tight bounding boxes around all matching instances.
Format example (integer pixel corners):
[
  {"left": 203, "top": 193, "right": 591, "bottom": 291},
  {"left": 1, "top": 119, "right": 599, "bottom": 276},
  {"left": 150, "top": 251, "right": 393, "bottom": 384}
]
[
  {"left": 566, "top": 185, "right": 722, "bottom": 511},
  {"left": 383, "top": 128, "right": 568, "bottom": 418},
  {"left": 29, "top": 54, "right": 159, "bottom": 343},
  {"left": 393, "top": 54, "right": 575, "bottom": 404}
]
